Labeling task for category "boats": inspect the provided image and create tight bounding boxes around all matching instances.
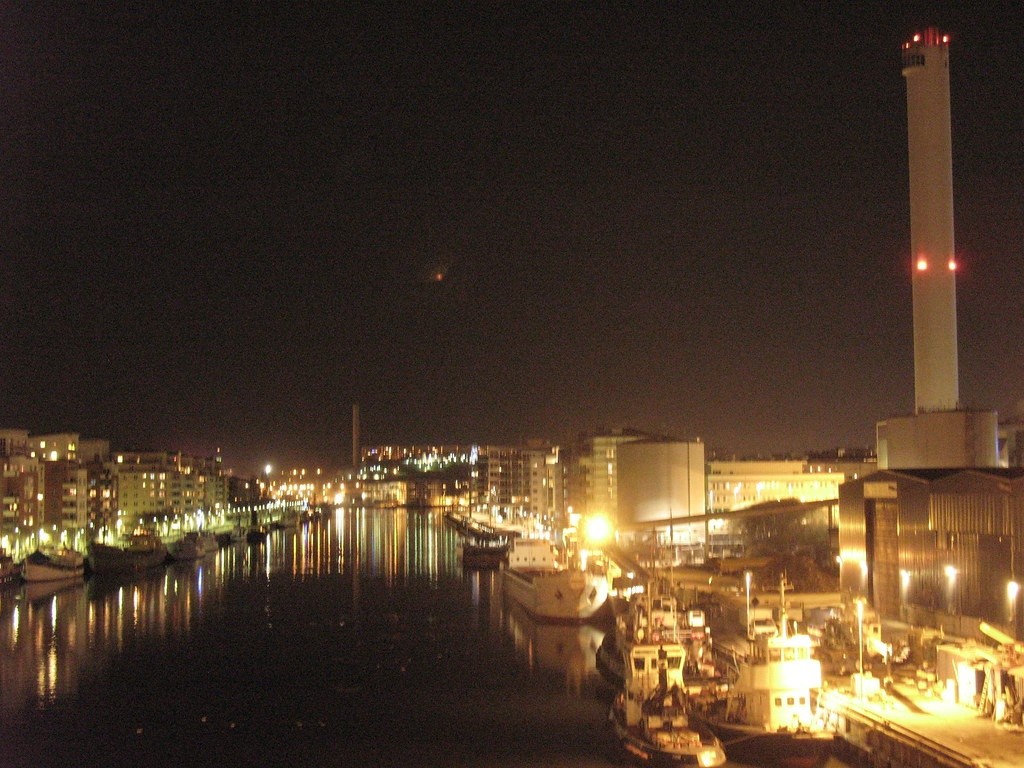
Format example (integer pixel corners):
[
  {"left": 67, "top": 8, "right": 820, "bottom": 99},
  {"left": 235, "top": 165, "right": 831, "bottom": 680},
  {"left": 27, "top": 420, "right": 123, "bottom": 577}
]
[
  {"left": 0, "top": 488, "right": 310, "bottom": 606},
  {"left": 443, "top": 473, "right": 836, "bottom": 767}
]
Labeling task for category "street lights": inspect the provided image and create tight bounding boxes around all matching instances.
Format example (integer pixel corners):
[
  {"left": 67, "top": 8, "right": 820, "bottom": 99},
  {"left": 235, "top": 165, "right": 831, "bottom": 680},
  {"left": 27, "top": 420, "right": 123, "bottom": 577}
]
[
  {"left": 745, "top": 570, "right": 751, "bottom": 638},
  {"left": 856, "top": 600, "right": 865, "bottom": 708}
]
